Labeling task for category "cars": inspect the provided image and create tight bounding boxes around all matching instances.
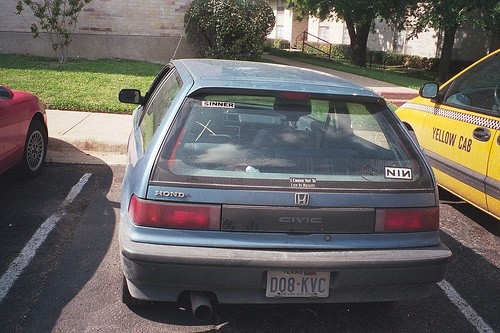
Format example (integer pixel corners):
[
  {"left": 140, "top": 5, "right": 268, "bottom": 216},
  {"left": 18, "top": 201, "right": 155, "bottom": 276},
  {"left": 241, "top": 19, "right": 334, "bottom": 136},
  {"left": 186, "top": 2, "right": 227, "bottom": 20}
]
[
  {"left": 394, "top": 49, "right": 500, "bottom": 221},
  {"left": 117, "top": 58, "right": 453, "bottom": 322},
  {"left": 0, "top": 84, "right": 49, "bottom": 178}
]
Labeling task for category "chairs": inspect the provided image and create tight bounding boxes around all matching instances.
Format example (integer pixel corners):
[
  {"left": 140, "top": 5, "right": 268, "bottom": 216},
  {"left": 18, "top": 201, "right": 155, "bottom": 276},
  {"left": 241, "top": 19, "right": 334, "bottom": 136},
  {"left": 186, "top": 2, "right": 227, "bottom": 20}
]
[
  {"left": 182, "top": 107, "right": 227, "bottom": 145},
  {"left": 253, "top": 93, "right": 311, "bottom": 147}
]
